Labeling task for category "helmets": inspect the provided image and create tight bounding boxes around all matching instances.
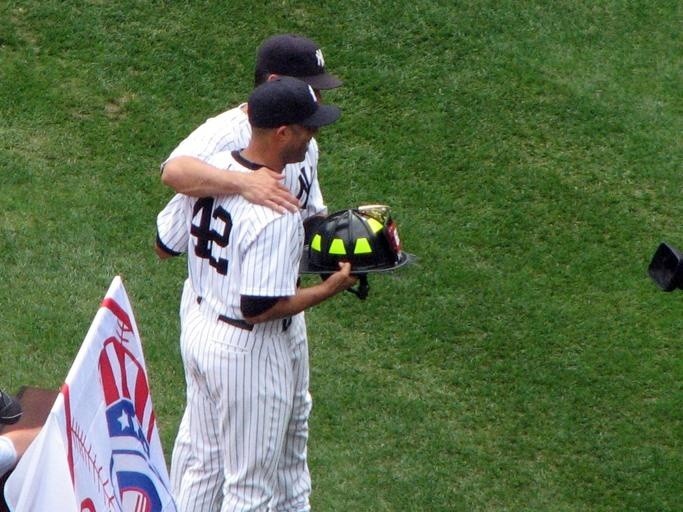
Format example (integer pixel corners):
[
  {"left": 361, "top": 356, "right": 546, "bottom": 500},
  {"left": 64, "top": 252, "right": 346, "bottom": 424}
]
[{"left": 299, "top": 210, "right": 409, "bottom": 273}]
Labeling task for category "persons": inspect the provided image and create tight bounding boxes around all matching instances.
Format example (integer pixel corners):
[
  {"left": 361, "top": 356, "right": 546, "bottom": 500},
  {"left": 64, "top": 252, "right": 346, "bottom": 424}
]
[
  {"left": 157, "top": 33, "right": 342, "bottom": 512},
  {"left": 0, "top": 425, "right": 43, "bottom": 479},
  {"left": 154, "top": 75, "right": 360, "bottom": 512}
]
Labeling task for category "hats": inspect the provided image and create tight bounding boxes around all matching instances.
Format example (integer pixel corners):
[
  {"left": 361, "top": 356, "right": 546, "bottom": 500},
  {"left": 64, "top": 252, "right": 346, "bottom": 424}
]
[
  {"left": 255, "top": 35, "right": 342, "bottom": 89},
  {"left": 248, "top": 77, "right": 341, "bottom": 127}
]
